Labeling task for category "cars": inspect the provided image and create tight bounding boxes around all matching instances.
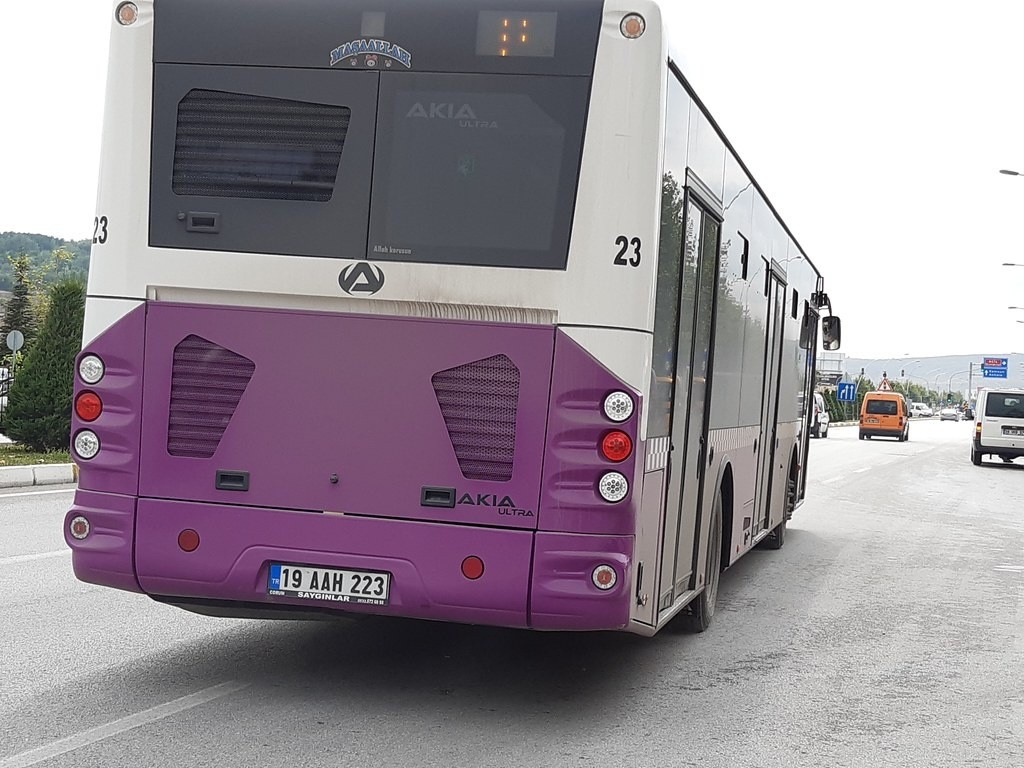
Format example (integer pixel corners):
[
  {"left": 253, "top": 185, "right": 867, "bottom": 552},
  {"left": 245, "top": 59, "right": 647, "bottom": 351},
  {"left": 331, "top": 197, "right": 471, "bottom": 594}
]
[{"left": 935, "top": 402, "right": 962, "bottom": 422}]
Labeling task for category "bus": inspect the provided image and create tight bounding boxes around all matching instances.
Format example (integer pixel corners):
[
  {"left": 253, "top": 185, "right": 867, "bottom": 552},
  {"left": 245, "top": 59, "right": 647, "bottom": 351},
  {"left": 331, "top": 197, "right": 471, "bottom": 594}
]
[{"left": 65, "top": 0, "right": 840, "bottom": 638}]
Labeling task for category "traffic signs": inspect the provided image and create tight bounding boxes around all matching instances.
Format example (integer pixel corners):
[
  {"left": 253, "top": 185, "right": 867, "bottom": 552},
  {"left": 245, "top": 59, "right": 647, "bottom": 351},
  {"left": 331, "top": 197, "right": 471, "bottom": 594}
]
[{"left": 983, "top": 369, "right": 1007, "bottom": 378}]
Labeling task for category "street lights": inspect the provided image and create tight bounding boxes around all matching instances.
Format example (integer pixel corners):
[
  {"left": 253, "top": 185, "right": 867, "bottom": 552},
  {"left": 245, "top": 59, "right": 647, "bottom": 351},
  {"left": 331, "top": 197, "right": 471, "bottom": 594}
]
[{"left": 852, "top": 354, "right": 946, "bottom": 420}]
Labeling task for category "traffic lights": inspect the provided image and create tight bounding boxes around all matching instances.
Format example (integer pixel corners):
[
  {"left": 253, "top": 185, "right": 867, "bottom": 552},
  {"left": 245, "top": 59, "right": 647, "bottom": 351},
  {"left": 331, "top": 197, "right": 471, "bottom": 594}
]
[
  {"left": 981, "top": 364, "right": 984, "bottom": 372},
  {"left": 947, "top": 394, "right": 951, "bottom": 402},
  {"left": 901, "top": 370, "right": 904, "bottom": 378}
]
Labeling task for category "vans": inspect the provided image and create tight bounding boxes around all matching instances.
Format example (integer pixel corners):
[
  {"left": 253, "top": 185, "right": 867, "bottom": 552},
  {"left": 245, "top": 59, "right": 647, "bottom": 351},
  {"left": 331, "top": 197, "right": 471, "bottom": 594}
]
[
  {"left": 859, "top": 392, "right": 912, "bottom": 442},
  {"left": 965, "top": 388, "right": 1024, "bottom": 466},
  {"left": 905, "top": 402, "right": 931, "bottom": 418},
  {"left": 809, "top": 392, "right": 832, "bottom": 438}
]
